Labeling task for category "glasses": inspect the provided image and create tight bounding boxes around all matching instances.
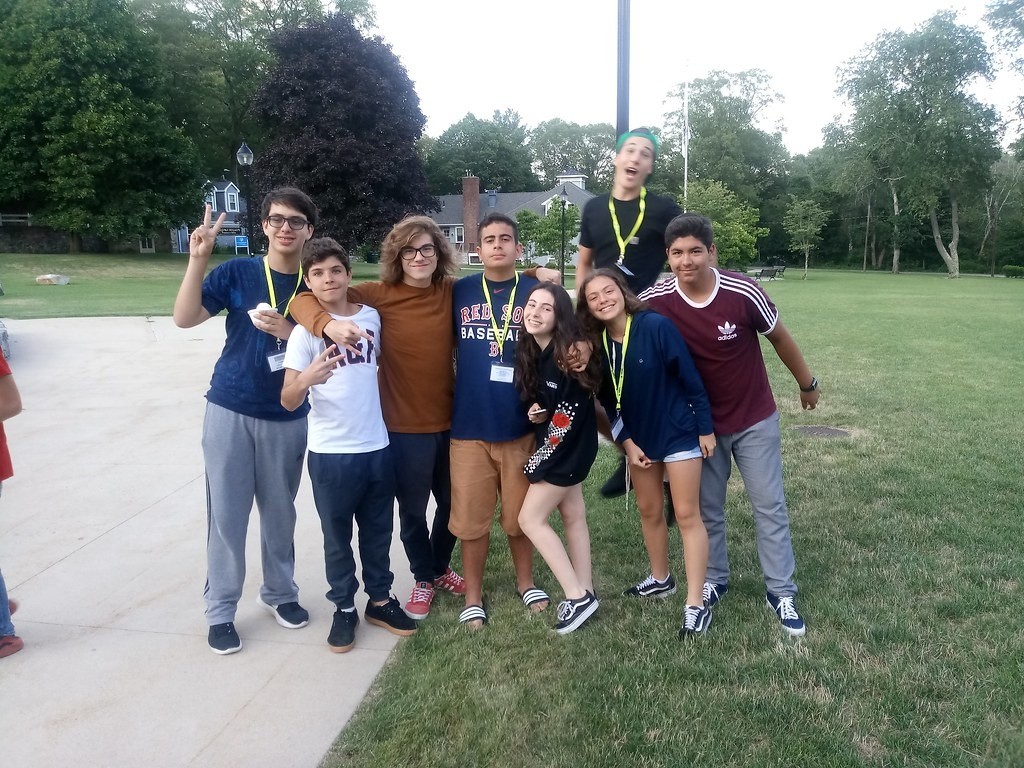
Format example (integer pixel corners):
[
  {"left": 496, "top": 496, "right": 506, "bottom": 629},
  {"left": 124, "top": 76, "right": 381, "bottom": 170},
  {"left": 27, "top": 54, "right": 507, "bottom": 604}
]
[
  {"left": 400, "top": 244, "right": 437, "bottom": 260},
  {"left": 266, "top": 215, "right": 309, "bottom": 230}
]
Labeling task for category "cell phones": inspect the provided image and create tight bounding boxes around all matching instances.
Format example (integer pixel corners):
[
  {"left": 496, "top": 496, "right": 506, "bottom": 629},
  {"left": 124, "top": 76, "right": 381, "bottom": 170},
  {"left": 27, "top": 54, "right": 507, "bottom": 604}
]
[{"left": 530, "top": 409, "right": 547, "bottom": 415}]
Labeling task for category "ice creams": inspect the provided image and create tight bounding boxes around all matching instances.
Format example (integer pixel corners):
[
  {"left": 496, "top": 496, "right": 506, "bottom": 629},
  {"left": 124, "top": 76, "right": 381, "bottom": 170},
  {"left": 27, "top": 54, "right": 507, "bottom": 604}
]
[{"left": 255, "top": 302, "right": 272, "bottom": 310}]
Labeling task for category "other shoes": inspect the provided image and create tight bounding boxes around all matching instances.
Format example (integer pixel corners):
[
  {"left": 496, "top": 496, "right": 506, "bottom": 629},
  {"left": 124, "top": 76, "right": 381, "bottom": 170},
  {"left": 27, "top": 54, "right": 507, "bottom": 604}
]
[
  {"left": 0, "top": 636, "right": 23, "bottom": 658},
  {"left": 8, "top": 600, "right": 17, "bottom": 615}
]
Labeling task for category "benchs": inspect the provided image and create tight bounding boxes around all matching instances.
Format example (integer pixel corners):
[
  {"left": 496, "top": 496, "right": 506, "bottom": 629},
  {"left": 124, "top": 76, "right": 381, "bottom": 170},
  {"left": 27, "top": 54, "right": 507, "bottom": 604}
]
[
  {"left": 755, "top": 269, "right": 778, "bottom": 281},
  {"left": 774, "top": 266, "right": 785, "bottom": 277}
]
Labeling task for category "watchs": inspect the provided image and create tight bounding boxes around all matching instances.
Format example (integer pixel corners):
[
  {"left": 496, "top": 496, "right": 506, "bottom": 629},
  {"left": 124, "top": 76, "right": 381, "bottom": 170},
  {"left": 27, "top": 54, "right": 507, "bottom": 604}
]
[{"left": 799, "top": 377, "right": 818, "bottom": 392}]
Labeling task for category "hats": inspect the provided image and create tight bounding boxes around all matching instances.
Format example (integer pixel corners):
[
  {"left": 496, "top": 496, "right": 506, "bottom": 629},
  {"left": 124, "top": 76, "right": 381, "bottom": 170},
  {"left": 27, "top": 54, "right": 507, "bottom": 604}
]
[{"left": 616, "top": 126, "right": 657, "bottom": 185}]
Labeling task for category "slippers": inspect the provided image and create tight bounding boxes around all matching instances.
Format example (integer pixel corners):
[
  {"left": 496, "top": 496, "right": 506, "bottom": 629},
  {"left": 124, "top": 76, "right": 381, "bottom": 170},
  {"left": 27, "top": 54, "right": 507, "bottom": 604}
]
[
  {"left": 459, "top": 600, "right": 486, "bottom": 633},
  {"left": 516, "top": 586, "right": 550, "bottom": 613}
]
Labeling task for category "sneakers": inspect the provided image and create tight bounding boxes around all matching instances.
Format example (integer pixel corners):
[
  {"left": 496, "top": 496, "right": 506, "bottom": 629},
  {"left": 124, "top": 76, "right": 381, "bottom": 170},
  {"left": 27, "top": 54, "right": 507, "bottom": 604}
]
[
  {"left": 256, "top": 593, "right": 310, "bottom": 628},
  {"left": 678, "top": 603, "right": 712, "bottom": 643},
  {"left": 364, "top": 593, "right": 419, "bottom": 636},
  {"left": 433, "top": 567, "right": 466, "bottom": 596},
  {"left": 403, "top": 582, "right": 435, "bottom": 620},
  {"left": 766, "top": 591, "right": 805, "bottom": 636},
  {"left": 550, "top": 588, "right": 599, "bottom": 637},
  {"left": 662, "top": 480, "right": 675, "bottom": 526},
  {"left": 701, "top": 581, "right": 728, "bottom": 608},
  {"left": 207, "top": 622, "right": 242, "bottom": 655},
  {"left": 326, "top": 608, "right": 360, "bottom": 653},
  {"left": 601, "top": 455, "right": 633, "bottom": 499},
  {"left": 622, "top": 573, "right": 676, "bottom": 602}
]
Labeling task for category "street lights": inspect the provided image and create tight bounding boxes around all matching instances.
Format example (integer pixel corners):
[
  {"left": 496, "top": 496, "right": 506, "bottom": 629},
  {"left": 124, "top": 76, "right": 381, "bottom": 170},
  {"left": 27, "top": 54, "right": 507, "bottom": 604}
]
[
  {"left": 236, "top": 141, "right": 257, "bottom": 258},
  {"left": 558, "top": 185, "right": 569, "bottom": 288}
]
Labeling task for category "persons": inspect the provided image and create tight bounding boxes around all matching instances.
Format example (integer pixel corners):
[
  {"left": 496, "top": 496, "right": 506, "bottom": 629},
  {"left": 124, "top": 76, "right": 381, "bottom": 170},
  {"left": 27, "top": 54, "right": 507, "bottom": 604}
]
[
  {"left": 280, "top": 235, "right": 418, "bottom": 653},
  {"left": 173, "top": 188, "right": 318, "bottom": 654},
  {"left": 0, "top": 344, "right": 25, "bottom": 656},
  {"left": 634, "top": 214, "right": 821, "bottom": 636},
  {"left": 448, "top": 212, "right": 593, "bottom": 631},
  {"left": 516, "top": 279, "right": 598, "bottom": 635},
  {"left": 288, "top": 214, "right": 561, "bottom": 620},
  {"left": 574, "top": 126, "right": 684, "bottom": 529},
  {"left": 575, "top": 268, "right": 718, "bottom": 640}
]
